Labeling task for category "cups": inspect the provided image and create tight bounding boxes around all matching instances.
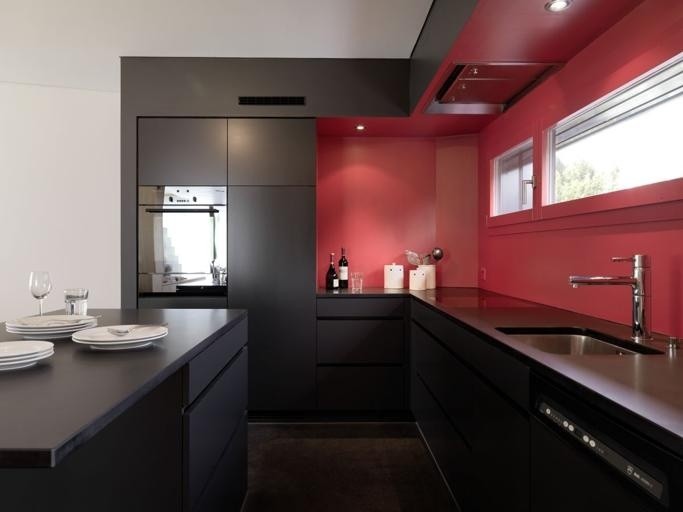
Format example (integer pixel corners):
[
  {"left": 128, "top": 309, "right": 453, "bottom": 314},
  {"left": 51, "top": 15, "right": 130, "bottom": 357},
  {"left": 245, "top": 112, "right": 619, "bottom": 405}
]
[
  {"left": 63, "top": 288, "right": 89, "bottom": 314},
  {"left": 351, "top": 272, "right": 363, "bottom": 291}
]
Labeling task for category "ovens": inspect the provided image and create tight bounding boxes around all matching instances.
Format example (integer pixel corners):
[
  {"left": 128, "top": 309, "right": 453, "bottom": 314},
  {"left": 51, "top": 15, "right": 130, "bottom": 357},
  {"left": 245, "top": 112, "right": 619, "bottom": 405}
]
[{"left": 136, "top": 185, "right": 227, "bottom": 296}]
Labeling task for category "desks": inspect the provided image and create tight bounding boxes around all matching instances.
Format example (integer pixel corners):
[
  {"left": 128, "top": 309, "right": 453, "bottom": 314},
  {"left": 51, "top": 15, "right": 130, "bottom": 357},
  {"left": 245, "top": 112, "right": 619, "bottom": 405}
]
[{"left": 0, "top": 309, "right": 248, "bottom": 512}]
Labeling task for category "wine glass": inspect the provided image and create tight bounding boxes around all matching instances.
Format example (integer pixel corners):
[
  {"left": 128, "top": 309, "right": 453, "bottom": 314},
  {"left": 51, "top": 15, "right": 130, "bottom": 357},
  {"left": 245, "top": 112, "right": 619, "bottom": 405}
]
[{"left": 29, "top": 271, "right": 53, "bottom": 315}]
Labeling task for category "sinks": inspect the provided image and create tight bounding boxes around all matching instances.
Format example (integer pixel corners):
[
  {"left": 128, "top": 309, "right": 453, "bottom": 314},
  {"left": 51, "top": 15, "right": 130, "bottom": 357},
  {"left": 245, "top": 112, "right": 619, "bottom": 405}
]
[{"left": 495, "top": 325, "right": 666, "bottom": 355}]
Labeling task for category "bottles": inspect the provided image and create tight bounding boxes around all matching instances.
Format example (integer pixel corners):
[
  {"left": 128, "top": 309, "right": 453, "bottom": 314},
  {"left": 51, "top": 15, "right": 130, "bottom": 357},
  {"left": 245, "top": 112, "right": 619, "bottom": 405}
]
[{"left": 325, "top": 247, "right": 349, "bottom": 292}]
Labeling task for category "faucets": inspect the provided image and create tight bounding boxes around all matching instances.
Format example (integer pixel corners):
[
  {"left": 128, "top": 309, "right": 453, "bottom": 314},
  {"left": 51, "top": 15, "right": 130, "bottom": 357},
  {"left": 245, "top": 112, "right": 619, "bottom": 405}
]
[{"left": 568, "top": 253, "right": 654, "bottom": 341}]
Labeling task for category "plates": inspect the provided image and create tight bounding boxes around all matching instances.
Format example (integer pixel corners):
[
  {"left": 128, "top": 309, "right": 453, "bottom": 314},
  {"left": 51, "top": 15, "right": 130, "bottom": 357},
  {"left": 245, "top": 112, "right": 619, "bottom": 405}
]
[{"left": 0, "top": 315, "right": 167, "bottom": 372}]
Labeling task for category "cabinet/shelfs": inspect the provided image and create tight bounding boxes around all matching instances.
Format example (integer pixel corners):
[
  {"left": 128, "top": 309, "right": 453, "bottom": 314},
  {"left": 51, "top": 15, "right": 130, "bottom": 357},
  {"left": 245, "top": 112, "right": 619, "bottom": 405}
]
[
  {"left": 408, "top": 296, "right": 531, "bottom": 511},
  {"left": 316, "top": 297, "right": 409, "bottom": 438},
  {"left": 139, "top": 117, "right": 316, "bottom": 436}
]
